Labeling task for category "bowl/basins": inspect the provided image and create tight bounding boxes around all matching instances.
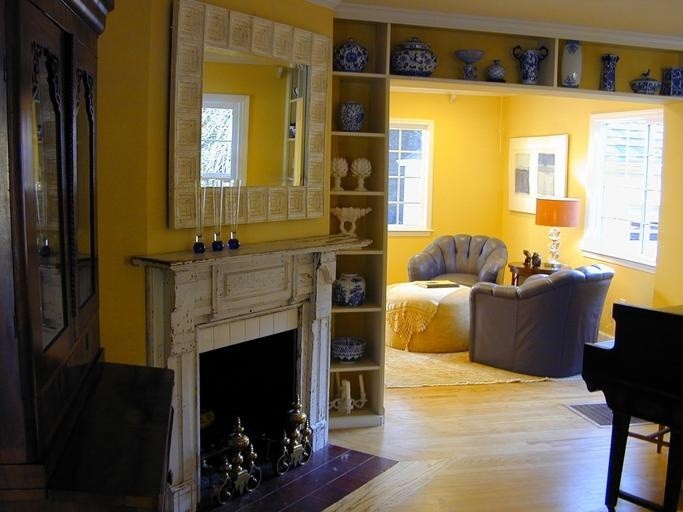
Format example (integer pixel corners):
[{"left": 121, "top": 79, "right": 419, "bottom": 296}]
[
  {"left": 628, "top": 81, "right": 662, "bottom": 95},
  {"left": 333, "top": 335, "right": 367, "bottom": 364}
]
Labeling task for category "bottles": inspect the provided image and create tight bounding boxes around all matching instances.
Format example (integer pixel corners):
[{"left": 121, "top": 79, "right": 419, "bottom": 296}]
[
  {"left": 562, "top": 39, "right": 581, "bottom": 88},
  {"left": 392, "top": 36, "right": 438, "bottom": 76},
  {"left": 333, "top": 272, "right": 365, "bottom": 307},
  {"left": 488, "top": 58, "right": 504, "bottom": 81},
  {"left": 513, "top": 41, "right": 547, "bottom": 85},
  {"left": 659, "top": 65, "right": 683, "bottom": 97},
  {"left": 333, "top": 37, "right": 369, "bottom": 73}
]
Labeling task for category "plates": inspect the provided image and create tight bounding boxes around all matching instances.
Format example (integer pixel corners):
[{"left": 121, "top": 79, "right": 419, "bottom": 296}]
[{"left": 333, "top": 237, "right": 373, "bottom": 250}]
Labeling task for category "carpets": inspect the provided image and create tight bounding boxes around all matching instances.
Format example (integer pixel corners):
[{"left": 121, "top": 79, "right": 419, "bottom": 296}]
[
  {"left": 382, "top": 340, "right": 585, "bottom": 382},
  {"left": 563, "top": 401, "right": 654, "bottom": 426}
]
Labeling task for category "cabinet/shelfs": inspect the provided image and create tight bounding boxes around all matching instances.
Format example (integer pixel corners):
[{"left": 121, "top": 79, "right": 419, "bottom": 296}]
[
  {"left": 3, "top": 0, "right": 105, "bottom": 469},
  {"left": 324, "top": 7, "right": 384, "bottom": 428}
]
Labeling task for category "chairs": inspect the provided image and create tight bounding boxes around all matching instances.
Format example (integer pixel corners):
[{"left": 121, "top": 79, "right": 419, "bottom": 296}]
[
  {"left": 404, "top": 232, "right": 615, "bottom": 377},
  {"left": 600, "top": 300, "right": 681, "bottom": 512}
]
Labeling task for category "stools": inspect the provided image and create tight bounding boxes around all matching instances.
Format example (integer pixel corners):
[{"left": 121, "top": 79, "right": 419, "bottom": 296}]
[{"left": 388, "top": 279, "right": 470, "bottom": 354}]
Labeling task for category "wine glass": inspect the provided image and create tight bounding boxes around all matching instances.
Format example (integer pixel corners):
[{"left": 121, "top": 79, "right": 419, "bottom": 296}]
[{"left": 454, "top": 49, "right": 485, "bottom": 80}]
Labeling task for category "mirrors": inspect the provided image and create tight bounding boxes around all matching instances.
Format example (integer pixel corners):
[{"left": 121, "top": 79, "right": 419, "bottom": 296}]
[{"left": 168, "top": 3, "right": 330, "bottom": 229}]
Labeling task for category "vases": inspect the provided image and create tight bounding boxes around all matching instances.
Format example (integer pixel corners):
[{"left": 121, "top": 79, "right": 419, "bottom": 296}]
[
  {"left": 509, "top": 43, "right": 550, "bottom": 84},
  {"left": 661, "top": 64, "right": 682, "bottom": 97},
  {"left": 338, "top": 99, "right": 368, "bottom": 133},
  {"left": 560, "top": 39, "right": 584, "bottom": 88},
  {"left": 486, "top": 59, "right": 507, "bottom": 83}
]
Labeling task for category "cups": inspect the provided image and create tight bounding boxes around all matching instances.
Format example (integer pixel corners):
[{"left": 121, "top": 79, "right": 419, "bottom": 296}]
[
  {"left": 192, "top": 179, "right": 241, "bottom": 252},
  {"left": 33, "top": 180, "right": 52, "bottom": 257}
]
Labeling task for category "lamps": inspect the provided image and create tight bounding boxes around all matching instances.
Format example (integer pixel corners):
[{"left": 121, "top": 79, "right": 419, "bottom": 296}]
[{"left": 534, "top": 196, "right": 580, "bottom": 268}]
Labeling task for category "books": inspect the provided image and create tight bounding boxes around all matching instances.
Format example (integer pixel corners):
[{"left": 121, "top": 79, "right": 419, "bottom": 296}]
[{"left": 414, "top": 280, "right": 459, "bottom": 288}]
